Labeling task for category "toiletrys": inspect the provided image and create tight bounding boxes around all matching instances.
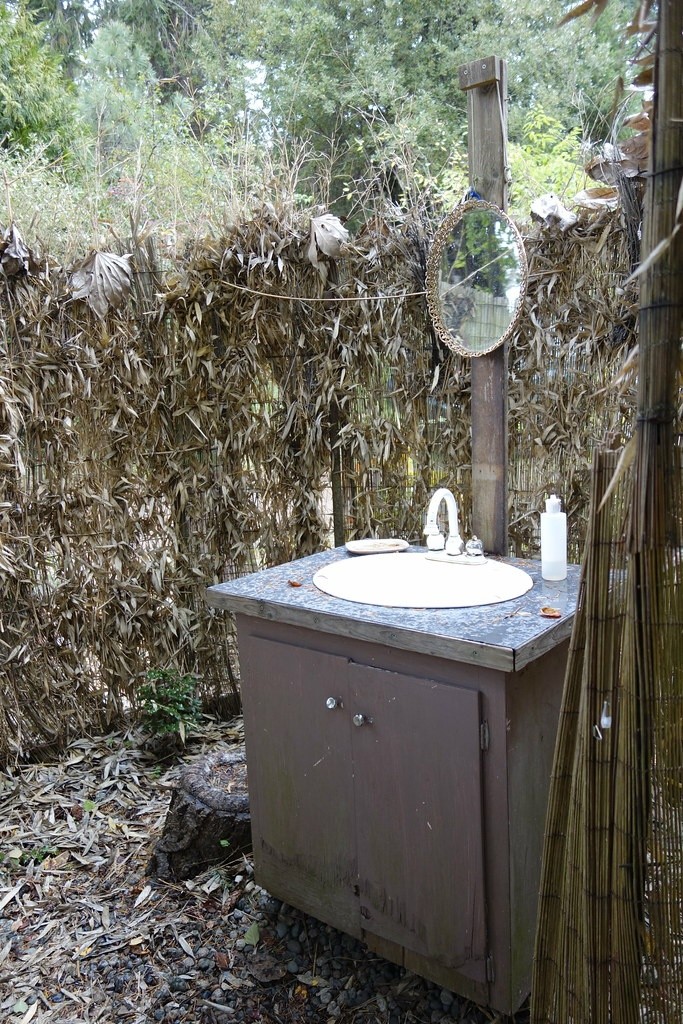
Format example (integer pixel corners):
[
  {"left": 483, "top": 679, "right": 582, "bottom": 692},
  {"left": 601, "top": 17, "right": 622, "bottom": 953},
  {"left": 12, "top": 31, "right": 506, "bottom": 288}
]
[{"left": 538, "top": 493, "right": 569, "bottom": 584}]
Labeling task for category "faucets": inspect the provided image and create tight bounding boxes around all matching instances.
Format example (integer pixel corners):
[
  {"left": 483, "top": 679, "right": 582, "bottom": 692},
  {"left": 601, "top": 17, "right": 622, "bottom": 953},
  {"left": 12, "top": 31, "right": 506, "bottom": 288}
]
[{"left": 423, "top": 487, "right": 467, "bottom": 555}]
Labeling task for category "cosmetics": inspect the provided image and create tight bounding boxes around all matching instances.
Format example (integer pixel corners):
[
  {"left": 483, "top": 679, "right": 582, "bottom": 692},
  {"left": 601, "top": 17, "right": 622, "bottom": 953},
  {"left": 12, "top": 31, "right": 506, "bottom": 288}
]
[{"left": 539, "top": 494, "right": 569, "bottom": 581}]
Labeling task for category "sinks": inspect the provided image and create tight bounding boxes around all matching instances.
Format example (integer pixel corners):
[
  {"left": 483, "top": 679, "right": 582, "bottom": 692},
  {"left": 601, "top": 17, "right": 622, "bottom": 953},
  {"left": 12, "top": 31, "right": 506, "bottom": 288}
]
[{"left": 311, "top": 548, "right": 534, "bottom": 612}]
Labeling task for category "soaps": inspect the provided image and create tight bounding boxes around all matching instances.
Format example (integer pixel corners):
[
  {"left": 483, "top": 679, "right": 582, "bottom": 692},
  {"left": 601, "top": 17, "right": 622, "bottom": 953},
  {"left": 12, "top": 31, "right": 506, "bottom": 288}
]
[{"left": 376, "top": 539, "right": 400, "bottom": 548}]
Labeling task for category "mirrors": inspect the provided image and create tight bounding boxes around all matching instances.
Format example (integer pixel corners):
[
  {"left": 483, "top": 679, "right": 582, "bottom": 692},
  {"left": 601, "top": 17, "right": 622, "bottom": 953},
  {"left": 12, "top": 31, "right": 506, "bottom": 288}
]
[{"left": 424, "top": 198, "right": 529, "bottom": 359}]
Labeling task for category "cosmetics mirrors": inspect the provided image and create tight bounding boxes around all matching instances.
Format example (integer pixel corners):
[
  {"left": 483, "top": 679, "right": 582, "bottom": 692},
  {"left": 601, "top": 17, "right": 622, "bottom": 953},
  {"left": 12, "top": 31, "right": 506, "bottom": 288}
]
[{"left": 426, "top": 197, "right": 526, "bottom": 359}]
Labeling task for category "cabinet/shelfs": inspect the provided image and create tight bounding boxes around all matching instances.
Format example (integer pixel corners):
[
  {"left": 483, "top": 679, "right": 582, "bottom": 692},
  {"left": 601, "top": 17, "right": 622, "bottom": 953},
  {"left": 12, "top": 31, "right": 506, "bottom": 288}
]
[{"left": 234, "top": 612, "right": 570, "bottom": 1018}]
